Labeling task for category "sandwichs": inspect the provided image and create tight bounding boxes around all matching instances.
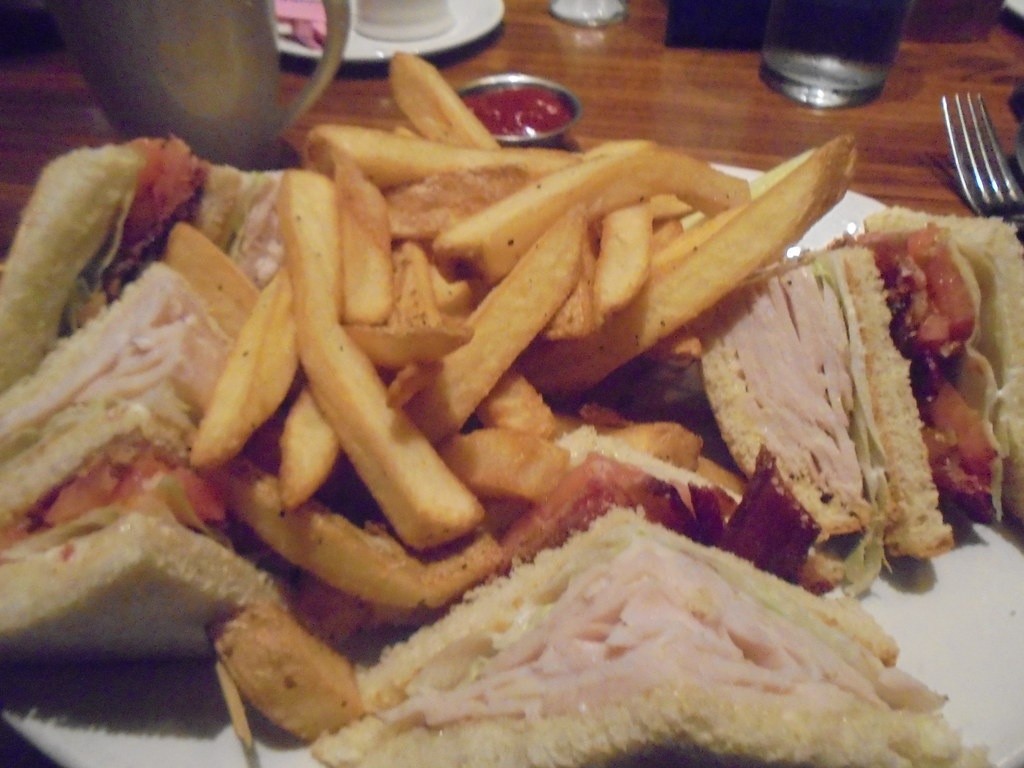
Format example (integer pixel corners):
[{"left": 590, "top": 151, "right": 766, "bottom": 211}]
[
  {"left": 297, "top": 424, "right": 995, "bottom": 767},
  {"left": 0, "top": 132, "right": 284, "bottom": 392},
  {"left": 0, "top": 259, "right": 373, "bottom": 664},
  {"left": 686, "top": 205, "right": 1024, "bottom": 574}
]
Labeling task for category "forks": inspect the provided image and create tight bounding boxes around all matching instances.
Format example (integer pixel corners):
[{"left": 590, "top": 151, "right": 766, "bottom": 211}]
[{"left": 940, "top": 92, "right": 1024, "bottom": 240}]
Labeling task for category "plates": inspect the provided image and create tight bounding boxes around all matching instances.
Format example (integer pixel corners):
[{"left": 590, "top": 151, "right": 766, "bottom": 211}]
[
  {"left": 0, "top": 160, "right": 1024, "bottom": 768},
  {"left": 275, "top": 0, "right": 504, "bottom": 63}
]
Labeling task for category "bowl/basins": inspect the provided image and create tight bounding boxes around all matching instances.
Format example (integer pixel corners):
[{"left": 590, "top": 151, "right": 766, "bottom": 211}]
[{"left": 457, "top": 74, "right": 583, "bottom": 146}]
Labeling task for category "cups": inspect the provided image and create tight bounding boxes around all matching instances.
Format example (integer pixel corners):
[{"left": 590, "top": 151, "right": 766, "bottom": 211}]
[
  {"left": 46, "top": 0, "right": 351, "bottom": 172},
  {"left": 548, "top": 0, "right": 630, "bottom": 28},
  {"left": 756, "top": 0, "right": 915, "bottom": 110},
  {"left": 351, "top": 0, "right": 456, "bottom": 42}
]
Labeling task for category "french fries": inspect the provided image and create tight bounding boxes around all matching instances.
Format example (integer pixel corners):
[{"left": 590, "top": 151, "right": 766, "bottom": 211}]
[{"left": 157, "top": 48, "right": 863, "bottom": 732}]
